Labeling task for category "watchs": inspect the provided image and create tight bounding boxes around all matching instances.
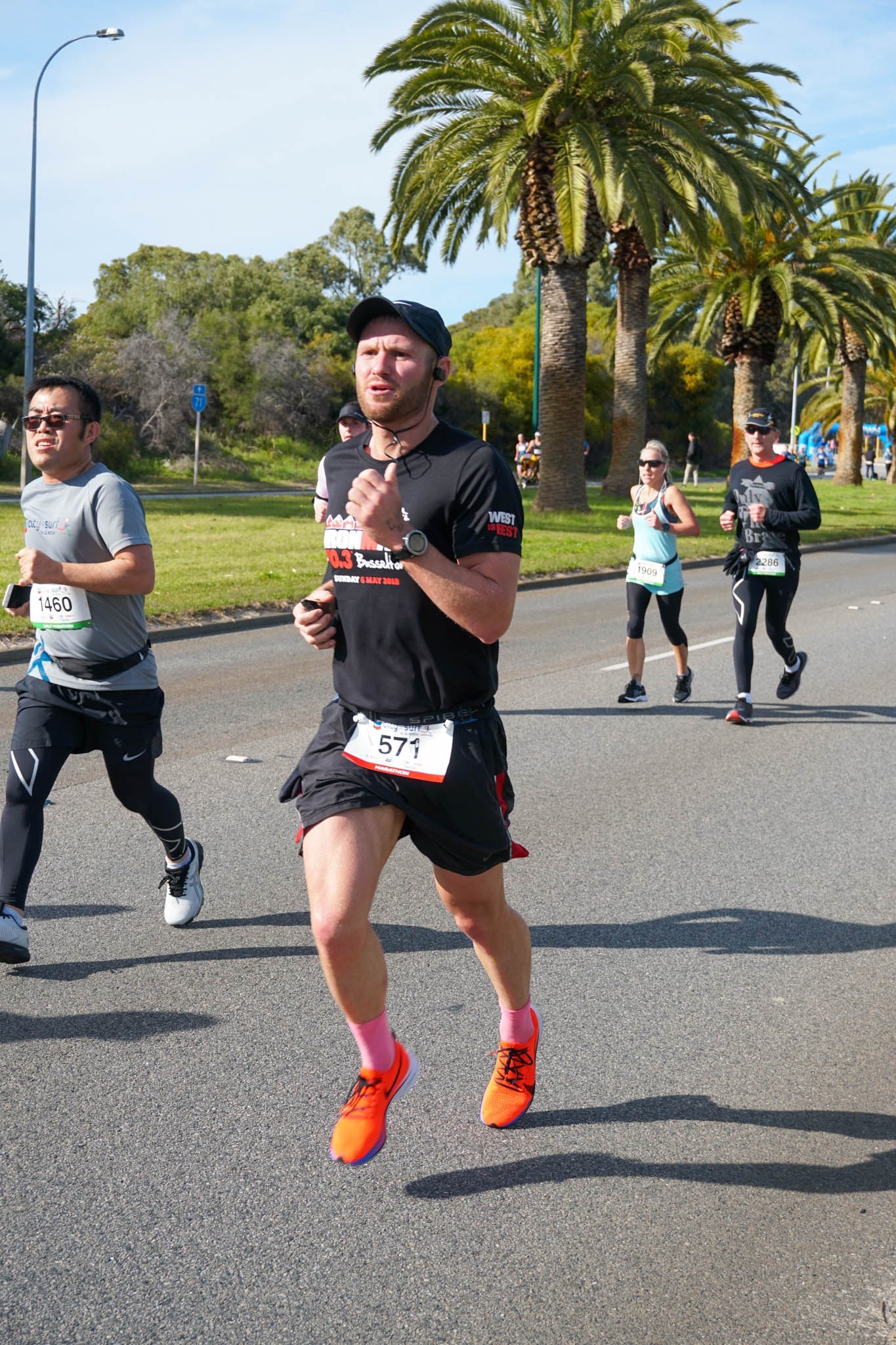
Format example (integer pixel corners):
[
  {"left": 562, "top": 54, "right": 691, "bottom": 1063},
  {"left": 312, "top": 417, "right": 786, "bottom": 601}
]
[
  {"left": 390, "top": 528, "right": 428, "bottom": 565},
  {"left": 662, "top": 523, "right": 670, "bottom": 532}
]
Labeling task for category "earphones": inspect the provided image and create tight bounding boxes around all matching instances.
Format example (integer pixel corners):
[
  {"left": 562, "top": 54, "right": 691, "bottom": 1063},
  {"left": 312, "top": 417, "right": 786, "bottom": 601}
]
[
  {"left": 664, "top": 466, "right": 667, "bottom": 472},
  {"left": 433, "top": 366, "right": 445, "bottom": 380}
]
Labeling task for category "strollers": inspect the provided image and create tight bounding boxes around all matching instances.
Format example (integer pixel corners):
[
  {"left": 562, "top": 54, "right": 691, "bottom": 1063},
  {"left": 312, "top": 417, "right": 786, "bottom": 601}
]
[{"left": 518, "top": 452, "right": 541, "bottom": 490}]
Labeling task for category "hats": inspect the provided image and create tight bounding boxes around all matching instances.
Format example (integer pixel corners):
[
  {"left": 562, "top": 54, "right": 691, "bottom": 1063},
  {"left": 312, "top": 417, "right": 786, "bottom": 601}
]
[
  {"left": 346, "top": 296, "right": 453, "bottom": 357},
  {"left": 747, "top": 407, "right": 779, "bottom": 430},
  {"left": 336, "top": 406, "right": 367, "bottom": 423}
]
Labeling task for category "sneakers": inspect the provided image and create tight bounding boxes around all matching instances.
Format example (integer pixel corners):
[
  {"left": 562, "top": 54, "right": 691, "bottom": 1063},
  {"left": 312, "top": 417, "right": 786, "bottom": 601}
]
[
  {"left": 328, "top": 1034, "right": 416, "bottom": 1169},
  {"left": 0, "top": 898, "right": 32, "bottom": 965},
  {"left": 618, "top": 680, "right": 648, "bottom": 704},
  {"left": 157, "top": 838, "right": 206, "bottom": 925},
  {"left": 776, "top": 651, "right": 808, "bottom": 699},
  {"left": 672, "top": 666, "right": 694, "bottom": 703},
  {"left": 479, "top": 1005, "right": 542, "bottom": 1130},
  {"left": 724, "top": 696, "right": 754, "bottom": 723}
]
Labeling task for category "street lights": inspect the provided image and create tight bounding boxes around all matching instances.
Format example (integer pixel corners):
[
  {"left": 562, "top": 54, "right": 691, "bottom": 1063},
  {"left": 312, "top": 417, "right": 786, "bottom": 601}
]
[{"left": 19, "top": 27, "right": 126, "bottom": 488}]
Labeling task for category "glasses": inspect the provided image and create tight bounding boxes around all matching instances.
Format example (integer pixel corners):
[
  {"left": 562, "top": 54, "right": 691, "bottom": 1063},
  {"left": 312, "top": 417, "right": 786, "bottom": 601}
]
[
  {"left": 21, "top": 413, "right": 94, "bottom": 430},
  {"left": 636, "top": 459, "right": 665, "bottom": 468},
  {"left": 746, "top": 427, "right": 772, "bottom": 435}
]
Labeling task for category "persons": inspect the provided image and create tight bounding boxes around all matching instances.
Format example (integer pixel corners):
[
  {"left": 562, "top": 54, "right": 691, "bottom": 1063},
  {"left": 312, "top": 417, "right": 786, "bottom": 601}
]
[
  {"left": 782, "top": 440, "right": 893, "bottom": 483},
  {"left": 512, "top": 431, "right": 542, "bottom": 484},
  {"left": 719, "top": 407, "right": 822, "bottom": 727},
  {"left": 681, "top": 432, "right": 702, "bottom": 488},
  {"left": 279, "top": 295, "right": 544, "bottom": 1168},
  {"left": 582, "top": 441, "right": 589, "bottom": 456},
  {"left": 617, "top": 440, "right": 695, "bottom": 704},
  {"left": 0, "top": 374, "right": 204, "bottom": 965},
  {"left": 313, "top": 402, "right": 368, "bottom": 524}
]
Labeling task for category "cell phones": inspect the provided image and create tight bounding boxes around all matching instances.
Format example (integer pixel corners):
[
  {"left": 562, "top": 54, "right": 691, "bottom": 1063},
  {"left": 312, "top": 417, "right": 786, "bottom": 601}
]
[{"left": 2, "top": 583, "right": 33, "bottom": 608}]
